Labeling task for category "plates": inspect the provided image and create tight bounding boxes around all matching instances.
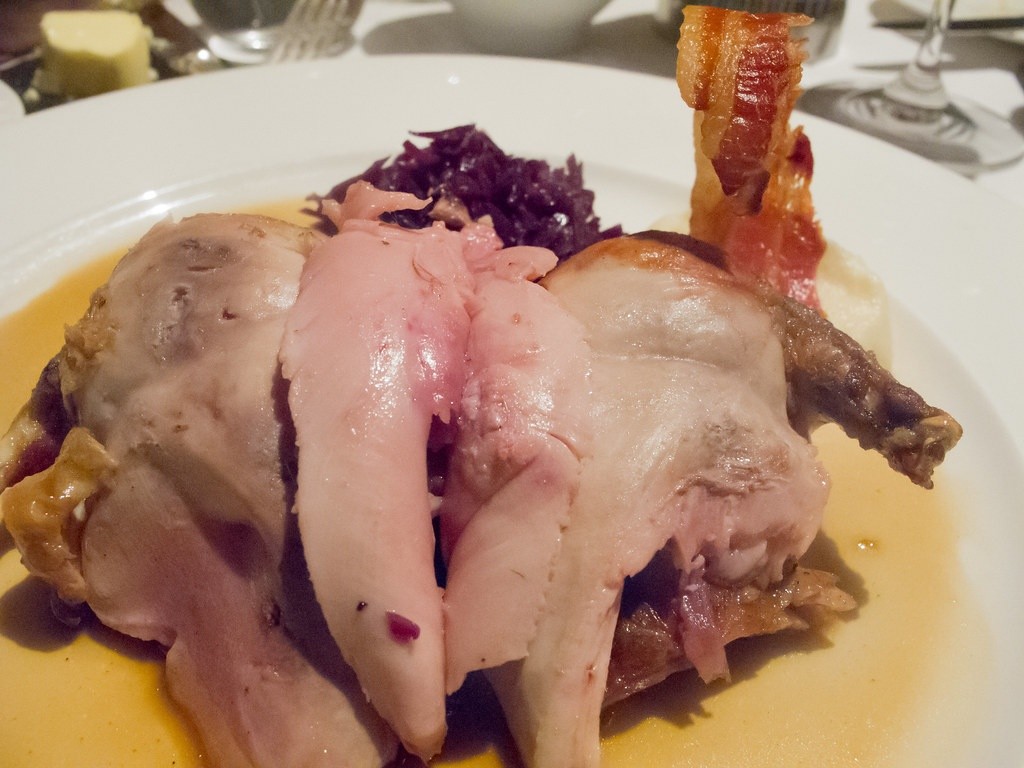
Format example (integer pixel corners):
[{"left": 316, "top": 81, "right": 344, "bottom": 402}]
[{"left": 1, "top": 48, "right": 1024, "bottom": 768}]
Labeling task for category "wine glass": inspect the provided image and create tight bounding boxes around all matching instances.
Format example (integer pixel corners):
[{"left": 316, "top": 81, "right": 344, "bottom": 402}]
[{"left": 791, "top": 0, "right": 1024, "bottom": 174}]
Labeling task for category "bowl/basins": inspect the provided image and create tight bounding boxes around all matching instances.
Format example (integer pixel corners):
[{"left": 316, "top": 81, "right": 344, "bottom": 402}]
[{"left": 449, "top": 0, "right": 614, "bottom": 58}]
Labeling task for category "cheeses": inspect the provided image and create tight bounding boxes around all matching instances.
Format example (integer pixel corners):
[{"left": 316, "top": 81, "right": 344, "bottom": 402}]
[{"left": 36, "top": 7, "right": 154, "bottom": 97}]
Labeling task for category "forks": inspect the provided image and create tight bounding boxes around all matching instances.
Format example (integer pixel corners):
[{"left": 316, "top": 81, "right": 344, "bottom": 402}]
[{"left": 264, "top": 0, "right": 359, "bottom": 64}]
